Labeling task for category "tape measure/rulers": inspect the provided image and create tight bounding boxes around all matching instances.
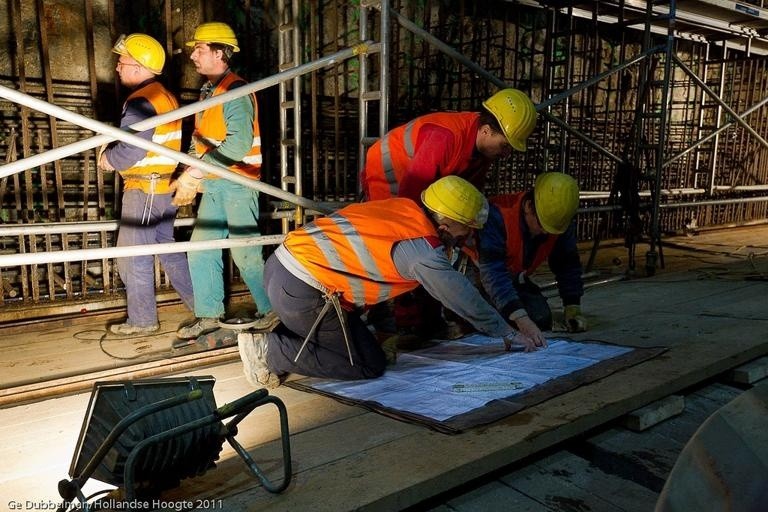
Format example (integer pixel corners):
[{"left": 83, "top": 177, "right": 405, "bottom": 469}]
[{"left": 450, "top": 382, "right": 524, "bottom": 392}]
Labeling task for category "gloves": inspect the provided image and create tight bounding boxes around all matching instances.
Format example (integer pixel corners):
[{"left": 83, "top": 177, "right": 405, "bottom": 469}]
[
  {"left": 552, "top": 305, "right": 589, "bottom": 332},
  {"left": 170, "top": 171, "right": 203, "bottom": 207}
]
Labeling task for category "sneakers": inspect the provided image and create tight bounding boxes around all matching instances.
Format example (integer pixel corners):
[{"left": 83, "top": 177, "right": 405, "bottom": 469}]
[
  {"left": 176, "top": 317, "right": 219, "bottom": 339},
  {"left": 238, "top": 331, "right": 281, "bottom": 390},
  {"left": 254, "top": 310, "right": 280, "bottom": 330},
  {"left": 110, "top": 323, "right": 160, "bottom": 336}
]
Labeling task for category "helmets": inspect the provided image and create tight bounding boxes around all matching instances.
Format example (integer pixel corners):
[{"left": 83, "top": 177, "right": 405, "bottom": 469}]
[
  {"left": 481, "top": 88, "right": 537, "bottom": 153},
  {"left": 186, "top": 21, "right": 240, "bottom": 53},
  {"left": 110, "top": 33, "right": 166, "bottom": 76},
  {"left": 534, "top": 172, "right": 580, "bottom": 236},
  {"left": 421, "top": 175, "right": 490, "bottom": 229}
]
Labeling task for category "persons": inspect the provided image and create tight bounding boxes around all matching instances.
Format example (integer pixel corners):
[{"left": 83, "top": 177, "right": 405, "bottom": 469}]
[
  {"left": 238, "top": 176, "right": 539, "bottom": 390},
  {"left": 165, "top": 22, "right": 271, "bottom": 340},
  {"left": 427, "top": 172, "right": 588, "bottom": 339},
  {"left": 358, "top": 88, "right": 538, "bottom": 350},
  {"left": 98, "top": 34, "right": 194, "bottom": 336}
]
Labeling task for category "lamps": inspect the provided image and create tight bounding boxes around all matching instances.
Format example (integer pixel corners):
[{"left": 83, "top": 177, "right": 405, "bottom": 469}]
[{"left": 48, "top": 375, "right": 295, "bottom": 511}]
